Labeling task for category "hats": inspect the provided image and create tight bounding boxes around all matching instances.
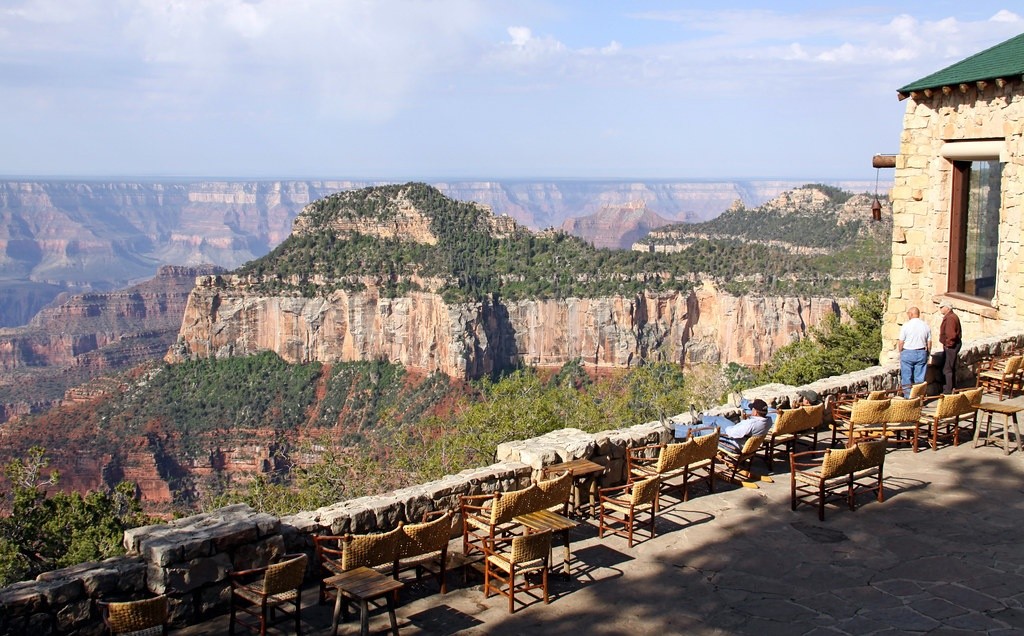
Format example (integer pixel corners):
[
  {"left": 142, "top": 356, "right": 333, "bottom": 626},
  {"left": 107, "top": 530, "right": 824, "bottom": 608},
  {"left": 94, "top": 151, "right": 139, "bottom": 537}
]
[
  {"left": 748, "top": 399, "right": 768, "bottom": 412},
  {"left": 935, "top": 299, "right": 954, "bottom": 308},
  {"left": 799, "top": 391, "right": 818, "bottom": 404}
]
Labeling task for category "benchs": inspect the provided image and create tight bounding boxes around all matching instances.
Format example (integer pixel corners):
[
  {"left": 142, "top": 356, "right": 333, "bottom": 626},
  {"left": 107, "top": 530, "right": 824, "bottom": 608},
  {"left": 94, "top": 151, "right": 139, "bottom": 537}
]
[
  {"left": 756, "top": 401, "right": 825, "bottom": 471},
  {"left": 317, "top": 503, "right": 456, "bottom": 610},
  {"left": 461, "top": 465, "right": 577, "bottom": 560},
  {"left": 921, "top": 386, "right": 987, "bottom": 451},
  {"left": 837, "top": 379, "right": 929, "bottom": 403},
  {"left": 623, "top": 424, "right": 724, "bottom": 502},
  {"left": 830, "top": 397, "right": 922, "bottom": 455},
  {"left": 788, "top": 435, "right": 889, "bottom": 522}
]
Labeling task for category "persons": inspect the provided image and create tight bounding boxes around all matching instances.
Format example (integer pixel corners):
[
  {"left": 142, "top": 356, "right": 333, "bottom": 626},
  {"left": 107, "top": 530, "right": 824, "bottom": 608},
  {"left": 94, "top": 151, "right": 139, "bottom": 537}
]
[
  {"left": 898, "top": 307, "right": 931, "bottom": 399},
  {"left": 659, "top": 404, "right": 772, "bottom": 454},
  {"left": 939, "top": 299, "right": 962, "bottom": 394},
  {"left": 732, "top": 387, "right": 818, "bottom": 435}
]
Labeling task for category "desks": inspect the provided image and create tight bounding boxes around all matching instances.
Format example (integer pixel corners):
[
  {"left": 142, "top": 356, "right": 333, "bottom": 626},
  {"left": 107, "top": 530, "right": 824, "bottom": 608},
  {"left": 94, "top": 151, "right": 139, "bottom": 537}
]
[
  {"left": 323, "top": 565, "right": 406, "bottom": 636},
  {"left": 511, "top": 509, "right": 582, "bottom": 584},
  {"left": 544, "top": 457, "right": 606, "bottom": 521}
]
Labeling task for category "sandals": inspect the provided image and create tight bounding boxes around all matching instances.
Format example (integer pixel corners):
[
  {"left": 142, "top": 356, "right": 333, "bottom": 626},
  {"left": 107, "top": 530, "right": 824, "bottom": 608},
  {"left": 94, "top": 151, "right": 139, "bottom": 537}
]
[{"left": 690, "top": 404, "right": 703, "bottom": 425}]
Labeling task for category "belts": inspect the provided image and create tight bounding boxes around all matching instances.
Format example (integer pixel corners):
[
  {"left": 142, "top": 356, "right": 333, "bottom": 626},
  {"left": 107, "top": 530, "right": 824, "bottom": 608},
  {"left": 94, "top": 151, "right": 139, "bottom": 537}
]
[{"left": 917, "top": 347, "right": 927, "bottom": 351}]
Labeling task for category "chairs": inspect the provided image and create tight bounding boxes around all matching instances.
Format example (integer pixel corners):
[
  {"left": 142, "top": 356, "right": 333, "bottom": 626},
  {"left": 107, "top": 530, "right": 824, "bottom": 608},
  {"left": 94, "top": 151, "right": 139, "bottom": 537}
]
[
  {"left": 718, "top": 432, "right": 768, "bottom": 481},
  {"left": 221, "top": 551, "right": 310, "bottom": 636},
  {"left": 479, "top": 527, "right": 554, "bottom": 614},
  {"left": 93, "top": 588, "right": 177, "bottom": 636},
  {"left": 597, "top": 471, "right": 662, "bottom": 549},
  {"left": 975, "top": 354, "right": 1023, "bottom": 400}
]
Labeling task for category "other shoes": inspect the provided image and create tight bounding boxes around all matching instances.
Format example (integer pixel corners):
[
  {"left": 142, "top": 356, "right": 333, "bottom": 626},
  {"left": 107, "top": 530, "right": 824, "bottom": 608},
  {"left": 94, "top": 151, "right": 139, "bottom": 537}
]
[
  {"left": 659, "top": 413, "right": 674, "bottom": 433},
  {"left": 733, "top": 390, "right": 744, "bottom": 408}
]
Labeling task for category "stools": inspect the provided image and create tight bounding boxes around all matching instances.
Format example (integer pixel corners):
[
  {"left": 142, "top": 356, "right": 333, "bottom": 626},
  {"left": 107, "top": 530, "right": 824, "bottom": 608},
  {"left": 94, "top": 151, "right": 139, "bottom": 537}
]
[{"left": 971, "top": 402, "right": 1024, "bottom": 455}]
